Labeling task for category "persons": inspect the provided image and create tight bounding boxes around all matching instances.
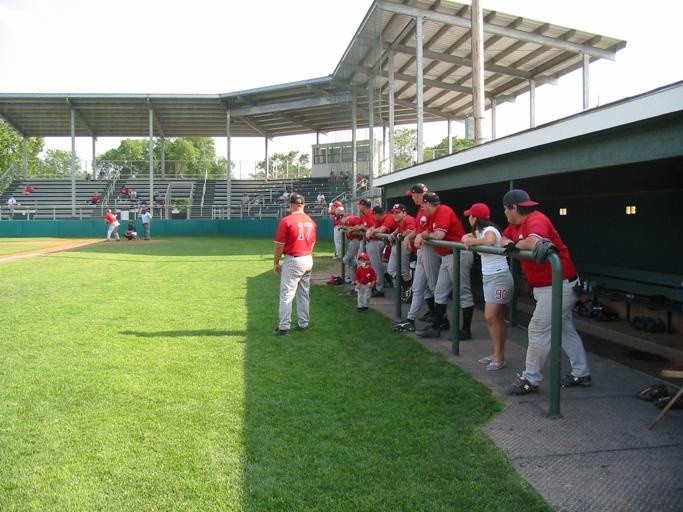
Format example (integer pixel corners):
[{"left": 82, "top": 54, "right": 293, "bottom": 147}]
[
  {"left": 22, "top": 183, "right": 33, "bottom": 195},
  {"left": 88, "top": 185, "right": 167, "bottom": 242},
  {"left": 4, "top": 194, "right": 17, "bottom": 205},
  {"left": 459, "top": 203, "right": 516, "bottom": 372},
  {"left": 498, "top": 189, "right": 594, "bottom": 395},
  {"left": 325, "top": 182, "right": 475, "bottom": 342},
  {"left": 271, "top": 192, "right": 318, "bottom": 335},
  {"left": 276, "top": 169, "right": 369, "bottom": 216}
]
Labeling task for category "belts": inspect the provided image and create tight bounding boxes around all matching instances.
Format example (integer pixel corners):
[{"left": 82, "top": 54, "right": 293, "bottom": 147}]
[
  {"left": 442, "top": 250, "right": 453, "bottom": 256},
  {"left": 568, "top": 276, "right": 578, "bottom": 283}
]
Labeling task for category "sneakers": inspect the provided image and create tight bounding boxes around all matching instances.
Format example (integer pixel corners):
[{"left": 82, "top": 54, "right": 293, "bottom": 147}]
[
  {"left": 560, "top": 373, "right": 591, "bottom": 387},
  {"left": 506, "top": 376, "right": 539, "bottom": 395},
  {"left": 419, "top": 312, "right": 436, "bottom": 321},
  {"left": 426, "top": 320, "right": 450, "bottom": 329},
  {"left": 392, "top": 321, "right": 416, "bottom": 332},
  {"left": 401, "top": 286, "right": 412, "bottom": 304},
  {"left": 654, "top": 394, "right": 682, "bottom": 409},
  {"left": 370, "top": 290, "right": 384, "bottom": 297},
  {"left": 426, "top": 315, "right": 442, "bottom": 323},
  {"left": 637, "top": 384, "right": 668, "bottom": 401},
  {"left": 416, "top": 326, "right": 441, "bottom": 337},
  {"left": 448, "top": 329, "right": 472, "bottom": 341},
  {"left": 293, "top": 323, "right": 305, "bottom": 330},
  {"left": 272, "top": 328, "right": 288, "bottom": 335}
]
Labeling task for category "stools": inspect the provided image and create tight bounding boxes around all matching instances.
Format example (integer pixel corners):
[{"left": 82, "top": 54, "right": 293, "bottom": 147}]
[{"left": 646, "top": 364, "right": 683, "bottom": 430}]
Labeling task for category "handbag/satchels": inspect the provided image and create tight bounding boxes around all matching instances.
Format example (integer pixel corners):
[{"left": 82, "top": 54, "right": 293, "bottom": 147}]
[{"left": 632, "top": 316, "right": 665, "bottom": 333}]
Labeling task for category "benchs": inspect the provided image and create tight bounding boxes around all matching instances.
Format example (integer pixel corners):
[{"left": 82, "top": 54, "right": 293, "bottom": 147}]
[{"left": 506, "top": 290, "right": 683, "bottom": 367}]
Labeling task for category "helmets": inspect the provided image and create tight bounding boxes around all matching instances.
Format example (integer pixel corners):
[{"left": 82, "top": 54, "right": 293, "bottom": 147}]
[{"left": 334, "top": 201, "right": 343, "bottom": 208}]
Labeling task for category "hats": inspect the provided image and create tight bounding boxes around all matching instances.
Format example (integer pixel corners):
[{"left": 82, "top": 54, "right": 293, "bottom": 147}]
[
  {"left": 358, "top": 253, "right": 369, "bottom": 260},
  {"left": 336, "top": 214, "right": 343, "bottom": 219},
  {"left": 423, "top": 193, "right": 440, "bottom": 202},
  {"left": 404, "top": 183, "right": 428, "bottom": 195},
  {"left": 356, "top": 198, "right": 366, "bottom": 205},
  {"left": 648, "top": 294, "right": 666, "bottom": 310},
  {"left": 503, "top": 189, "right": 539, "bottom": 207},
  {"left": 464, "top": 204, "right": 490, "bottom": 220},
  {"left": 373, "top": 206, "right": 384, "bottom": 214},
  {"left": 291, "top": 194, "right": 305, "bottom": 204},
  {"left": 390, "top": 204, "right": 406, "bottom": 213}
]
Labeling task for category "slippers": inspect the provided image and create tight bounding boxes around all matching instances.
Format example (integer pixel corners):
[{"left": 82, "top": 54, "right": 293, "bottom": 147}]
[
  {"left": 479, "top": 356, "right": 494, "bottom": 365},
  {"left": 486, "top": 361, "right": 507, "bottom": 371}
]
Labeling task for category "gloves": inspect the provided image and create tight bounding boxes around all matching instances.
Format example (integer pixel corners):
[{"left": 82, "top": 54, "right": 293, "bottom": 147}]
[
  {"left": 573, "top": 282, "right": 584, "bottom": 297},
  {"left": 503, "top": 242, "right": 520, "bottom": 254},
  {"left": 329, "top": 202, "right": 333, "bottom": 207}
]
